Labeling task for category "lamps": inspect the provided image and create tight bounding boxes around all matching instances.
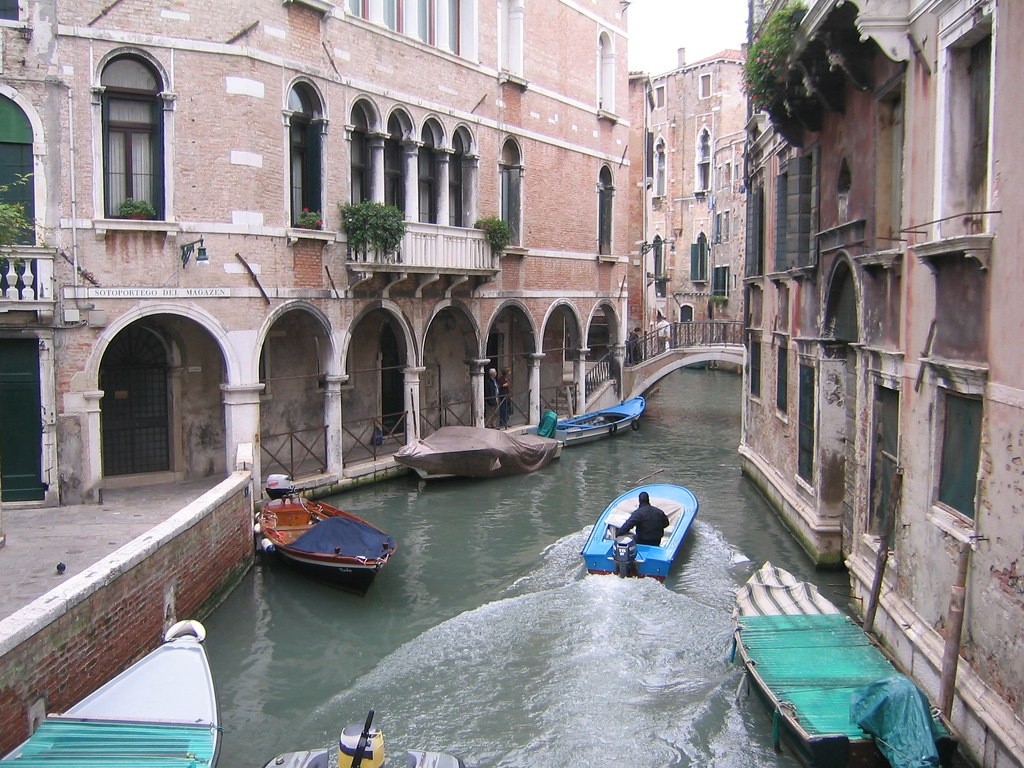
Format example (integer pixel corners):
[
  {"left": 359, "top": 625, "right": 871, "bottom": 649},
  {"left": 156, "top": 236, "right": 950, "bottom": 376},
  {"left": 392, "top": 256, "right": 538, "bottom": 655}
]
[
  {"left": 648, "top": 272, "right": 673, "bottom": 287},
  {"left": 641, "top": 237, "right": 677, "bottom": 256},
  {"left": 180, "top": 234, "right": 210, "bottom": 271}
]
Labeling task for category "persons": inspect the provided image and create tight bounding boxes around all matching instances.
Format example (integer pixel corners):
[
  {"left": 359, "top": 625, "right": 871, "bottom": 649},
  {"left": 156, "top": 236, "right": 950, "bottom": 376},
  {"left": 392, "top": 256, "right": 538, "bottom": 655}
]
[
  {"left": 616, "top": 492, "right": 669, "bottom": 546},
  {"left": 629, "top": 327, "right": 642, "bottom": 364},
  {"left": 484, "top": 367, "right": 512, "bottom": 428},
  {"left": 658, "top": 316, "right": 670, "bottom": 353}
]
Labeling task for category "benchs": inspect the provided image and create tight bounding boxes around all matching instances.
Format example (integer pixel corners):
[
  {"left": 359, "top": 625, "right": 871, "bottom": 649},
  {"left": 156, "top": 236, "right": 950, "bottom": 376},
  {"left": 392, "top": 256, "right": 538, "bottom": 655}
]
[
  {"left": 560, "top": 423, "right": 596, "bottom": 428},
  {"left": 277, "top": 524, "right": 315, "bottom": 531}
]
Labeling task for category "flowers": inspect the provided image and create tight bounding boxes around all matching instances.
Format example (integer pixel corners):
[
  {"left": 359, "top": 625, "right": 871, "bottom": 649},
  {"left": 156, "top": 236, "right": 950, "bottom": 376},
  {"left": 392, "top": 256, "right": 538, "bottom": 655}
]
[{"left": 295, "top": 207, "right": 323, "bottom": 230}]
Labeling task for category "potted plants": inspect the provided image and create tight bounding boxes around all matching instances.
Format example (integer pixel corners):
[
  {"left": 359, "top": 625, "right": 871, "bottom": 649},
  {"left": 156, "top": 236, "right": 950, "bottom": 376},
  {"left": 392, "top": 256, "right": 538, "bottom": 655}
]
[{"left": 117, "top": 198, "right": 156, "bottom": 221}]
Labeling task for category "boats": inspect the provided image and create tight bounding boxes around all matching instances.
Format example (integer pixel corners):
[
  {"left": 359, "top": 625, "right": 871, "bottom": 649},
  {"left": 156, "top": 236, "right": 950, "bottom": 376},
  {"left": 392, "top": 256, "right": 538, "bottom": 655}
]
[
  {"left": 258, "top": 708, "right": 462, "bottom": 768},
  {"left": 730, "top": 560, "right": 961, "bottom": 768},
  {"left": 1, "top": 616, "right": 224, "bottom": 767},
  {"left": 254, "top": 473, "right": 398, "bottom": 598},
  {"left": 579, "top": 482, "right": 701, "bottom": 584},
  {"left": 390, "top": 410, "right": 564, "bottom": 480},
  {"left": 526, "top": 396, "right": 647, "bottom": 447}
]
